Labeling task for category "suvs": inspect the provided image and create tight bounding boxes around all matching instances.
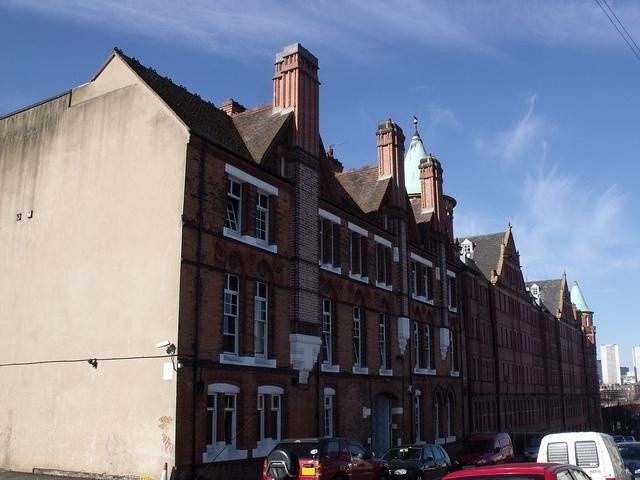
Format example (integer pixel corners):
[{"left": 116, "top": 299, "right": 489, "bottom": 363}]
[{"left": 263, "top": 435, "right": 391, "bottom": 480}]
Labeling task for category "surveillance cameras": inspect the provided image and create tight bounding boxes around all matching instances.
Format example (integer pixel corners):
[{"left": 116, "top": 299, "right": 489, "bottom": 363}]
[{"left": 157, "top": 341, "right": 171, "bottom": 348}]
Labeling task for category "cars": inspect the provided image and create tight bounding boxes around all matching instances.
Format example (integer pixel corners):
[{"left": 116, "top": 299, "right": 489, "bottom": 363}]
[
  {"left": 616, "top": 441, "right": 640, "bottom": 480},
  {"left": 381, "top": 444, "right": 452, "bottom": 480},
  {"left": 523, "top": 438, "right": 541, "bottom": 459},
  {"left": 440, "top": 462, "right": 592, "bottom": 480},
  {"left": 614, "top": 436, "right": 635, "bottom": 442}
]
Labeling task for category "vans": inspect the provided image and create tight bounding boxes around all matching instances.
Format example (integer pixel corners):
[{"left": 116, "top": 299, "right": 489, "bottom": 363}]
[
  {"left": 537, "top": 432, "right": 632, "bottom": 480},
  {"left": 453, "top": 431, "right": 514, "bottom": 469}
]
